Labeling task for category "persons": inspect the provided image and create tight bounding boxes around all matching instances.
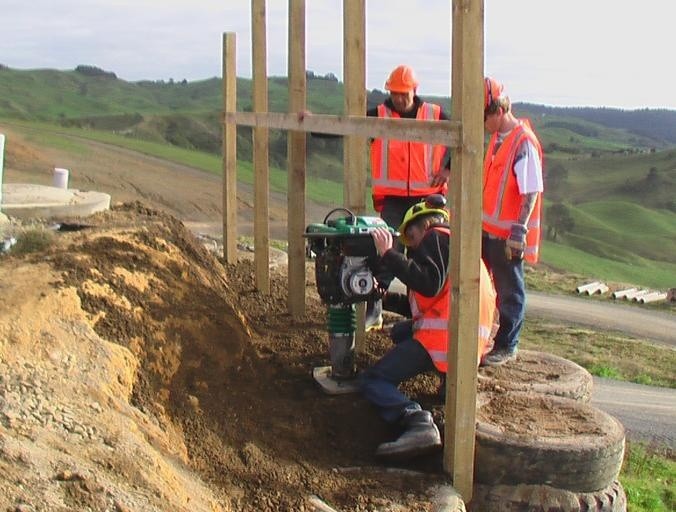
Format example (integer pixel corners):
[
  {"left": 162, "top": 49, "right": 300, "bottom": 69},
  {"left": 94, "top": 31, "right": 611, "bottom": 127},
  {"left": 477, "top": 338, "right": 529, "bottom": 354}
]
[
  {"left": 307, "top": 67, "right": 451, "bottom": 290},
  {"left": 481, "top": 76, "right": 545, "bottom": 366},
  {"left": 361, "top": 202, "right": 497, "bottom": 463}
]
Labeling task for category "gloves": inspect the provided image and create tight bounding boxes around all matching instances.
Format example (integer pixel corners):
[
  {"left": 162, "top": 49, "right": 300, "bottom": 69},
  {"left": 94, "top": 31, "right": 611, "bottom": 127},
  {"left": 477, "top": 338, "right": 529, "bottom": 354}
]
[{"left": 504, "top": 223, "right": 529, "bottom": 261}]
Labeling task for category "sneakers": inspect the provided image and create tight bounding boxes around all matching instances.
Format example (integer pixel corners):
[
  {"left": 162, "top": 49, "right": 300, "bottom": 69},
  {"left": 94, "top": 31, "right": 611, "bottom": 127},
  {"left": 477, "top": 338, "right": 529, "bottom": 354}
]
[{"left": 481, "top": 341, "right": 517, "bottom": 367}]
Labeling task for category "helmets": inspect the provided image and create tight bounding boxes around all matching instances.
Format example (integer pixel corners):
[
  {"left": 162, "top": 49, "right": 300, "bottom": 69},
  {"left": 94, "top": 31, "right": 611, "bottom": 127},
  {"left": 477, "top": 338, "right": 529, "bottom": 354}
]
[
  {"left": 483, "top": 77, "right": 504, "bottom": 110},
  {"left": 385, "top": 65, "right": 419, "bottom": 93},
  {"left": 398, "top": 199, "right": 449, "bottom": 246}
]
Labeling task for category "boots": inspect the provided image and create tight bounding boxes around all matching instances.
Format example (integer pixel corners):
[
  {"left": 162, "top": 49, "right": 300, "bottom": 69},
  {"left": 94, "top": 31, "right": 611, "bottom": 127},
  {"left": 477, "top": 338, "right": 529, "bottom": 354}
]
[{"left": 375, "top": 410, "right": 444, "bottom": 459}]
